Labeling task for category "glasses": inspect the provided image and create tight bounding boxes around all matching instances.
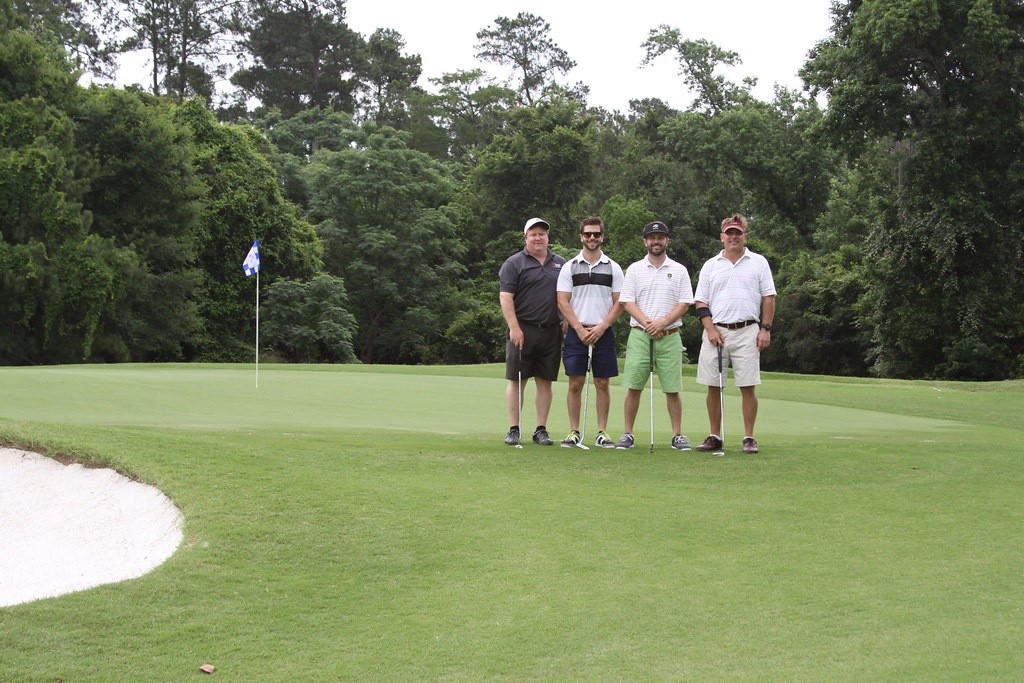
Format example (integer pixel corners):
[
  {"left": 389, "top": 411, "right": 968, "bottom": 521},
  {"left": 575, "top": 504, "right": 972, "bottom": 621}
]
[
  {"left": 582, "top": 232, "right": 602, "bottom": 238},
  {"left": 724, "top": 228, "right": 742, "bottom": 236}
]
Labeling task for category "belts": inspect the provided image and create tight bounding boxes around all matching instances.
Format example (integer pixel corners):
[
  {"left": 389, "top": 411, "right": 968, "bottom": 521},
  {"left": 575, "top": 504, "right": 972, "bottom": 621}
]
[
  {"left": 712, "top": 320, "right": 756, "bottom": 330},
  {"left": 527, "top": 323, "right": 555, "bottom": 329},
  {"left": 634, "top": 326, "right": 678, "bottom": 336}
]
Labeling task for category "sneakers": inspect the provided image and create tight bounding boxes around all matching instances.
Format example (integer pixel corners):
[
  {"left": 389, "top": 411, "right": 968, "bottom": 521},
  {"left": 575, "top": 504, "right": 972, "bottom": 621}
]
[
  {"left": 743, "top": 437, "right": 758, "bottom": 454},
  {"left": 533, "top": 428, "right": 553, "bottom": 445},
  {"left": 505, "top": 429, "right": 520, "bottom": 444},
  {"left": 696, "top": 436, "right": 722, "bottom": 452},
  {"left": 595, "top": 430, "right": 616, "bottom": 449},
  {"left": 615, "top": 433, "right": 635, "bottom": 449},
  {"left": 561, "top": 429, "right": 581, "bottom": 447},
  {"left": 671, "top": 434, "right": 692, "bottom": 451}
]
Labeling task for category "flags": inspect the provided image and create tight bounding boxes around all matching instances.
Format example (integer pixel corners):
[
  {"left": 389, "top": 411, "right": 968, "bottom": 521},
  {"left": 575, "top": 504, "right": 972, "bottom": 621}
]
[{"left": 242, "top": 240, "right": 261, "bottom": 277}]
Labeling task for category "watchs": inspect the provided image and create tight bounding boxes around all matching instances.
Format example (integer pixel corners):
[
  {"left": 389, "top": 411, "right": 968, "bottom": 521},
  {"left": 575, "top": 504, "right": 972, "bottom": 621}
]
[{"left": 761, "top": 323, "right": 772, "bottom": 331}]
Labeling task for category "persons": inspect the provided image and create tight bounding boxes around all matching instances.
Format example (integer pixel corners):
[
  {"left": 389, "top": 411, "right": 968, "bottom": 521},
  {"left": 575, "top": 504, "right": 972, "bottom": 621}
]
[
  {"left": 556, "top": 216, "right": 625, "bottom": 448},
  {"left": 694, "top": 214, "right": 779, "bottom": 453},
  {"left": 615, "top": 221, "right": 695, "bottom": 451},
  {"left": 498, "top": 217, "right": 569, "bottom": 445}
]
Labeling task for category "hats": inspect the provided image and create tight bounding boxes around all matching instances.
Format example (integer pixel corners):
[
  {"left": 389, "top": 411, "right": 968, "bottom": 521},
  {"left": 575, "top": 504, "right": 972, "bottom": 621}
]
[
  {"left": 721, "top": 220, "right": 745, "bottom": 233},
  {"left": 524, "top": 217, "right": 550, "bottom": 235},
  {"left": 644, "top": 223, "right": 669, "bottom": 238}
]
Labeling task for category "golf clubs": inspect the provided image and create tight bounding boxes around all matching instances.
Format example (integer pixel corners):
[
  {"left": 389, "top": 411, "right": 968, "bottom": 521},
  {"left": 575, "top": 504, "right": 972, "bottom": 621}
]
[
  {"left": 649, "top": 337, "right": 655, "bottom": 453},
  {"left": 576, "top": 344, "right": 593, "bottom": 451},
  {"left": 711, "top": 343, "right": 725, "bottom": 457},
  {"left": 514, "top": 349, "right": 524, "bottom": 448}
]
